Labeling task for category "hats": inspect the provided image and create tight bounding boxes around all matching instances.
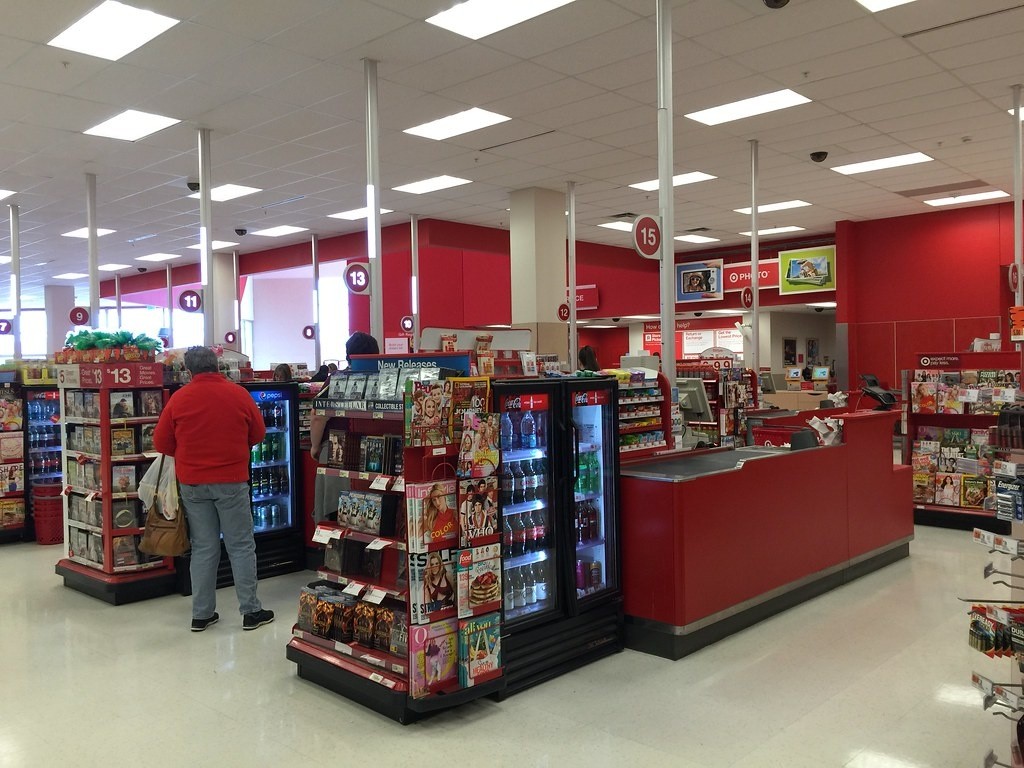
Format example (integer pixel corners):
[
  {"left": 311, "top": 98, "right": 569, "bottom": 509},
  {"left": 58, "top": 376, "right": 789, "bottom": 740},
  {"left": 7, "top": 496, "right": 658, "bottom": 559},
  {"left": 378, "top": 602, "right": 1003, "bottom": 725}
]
[{"left": 345, "top": 332, "right": 380, "bottom": 366}]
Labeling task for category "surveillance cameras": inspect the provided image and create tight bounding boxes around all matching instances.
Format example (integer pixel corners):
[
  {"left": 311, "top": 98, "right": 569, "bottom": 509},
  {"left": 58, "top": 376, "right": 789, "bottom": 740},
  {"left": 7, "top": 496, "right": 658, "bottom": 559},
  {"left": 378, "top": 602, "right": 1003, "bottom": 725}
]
[
  {"left": 810, "top": 152, "right": 828, "bottom": 162},
  {"left": 138, "top": 268, "right": 147, "bottom": 273},
  {"left": 187, "top": 183, "right": 200, "bottom": 192},
  {"left": 735, "top": 321, "right": 747, "bottom": 336},
  {"left": 612, "top": 318, "right": 620, "bottom": 322},
  {"left": 815, "top": 308, "right": 824, "bottom": 312},
  {"left": 763, "top": 0, "right": 790, "bottom": 9},
  {"left": 693, "top": 313, "right": 702, "bottom": 317},
  {"left": 235, "top": 229, "right": 247, "bottom": 236}
]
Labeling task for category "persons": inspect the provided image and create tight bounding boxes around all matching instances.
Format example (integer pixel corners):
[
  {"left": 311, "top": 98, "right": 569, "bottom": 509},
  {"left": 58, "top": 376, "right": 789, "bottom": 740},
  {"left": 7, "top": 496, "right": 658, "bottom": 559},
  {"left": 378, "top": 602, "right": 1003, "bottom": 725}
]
[
  {"left": 113, "top": 399, "right": 128, "bottom": 417},
  {"left": 926, "top": 374, "right": 931, "bottom": 382},
  {"left": 419, "top": 484, "right": 458, "bottom": 542},
  {"left": 345, "top": 331, "right": 379, "bottom": 366},
  {"left": 151, "top": 346, "right": 275, "bottom": 631},
  {"left": 421, "top": 397, "right": 441, "bottom": 425},
  {"left": 411, "top": 390, "right": 426, "bottom": 421},
  {"left": 940, "top": 475, "right": 959, "bottom": 505},
  {"left": 944, "top": 458, "right": 957, "bottom": 473},
  {"left": 309, "top": 363, "right": 337, "bottom": 382},
  {"left": 425, "top": 637, "right": 442, "bottom": 686},
  {"left": 430, "top": 384, "right": 450, "bottom": 411},
  {"left": 917, "top": 373, "right": 923, "bottom": 382},
  {"left": 685, "top": 272, "right": 704, "bottom": 291},
  {"left": 913, "top": 382, "right": 936, "bottom": 414},
  {"left": 467, "top": 494, "right": 496, "bottom": 537},
  {"left": 424, "top": 551, "right": 457, "bottom": 612},
  {"left": 8, "top": 466, "right": 20, "bottom": 492},
  {"left": 921, "top": 371, "right": 927, "bottom": 382},
  {"left": 932, "top": 374, "right": 936, "bottom": 382},
  {"left": 442, "top": 380, "right": 451, "bottom": 394},
  {"left": 1005, "top": 372, "right": 1014, "bottom": 383},
  {"left": 483, "top": 487, "right": 498, "bottom": 509},
  {"left": 473, "top": 479, "right": 487, "bottom": 508},
  {"left": 1015, "top": 372, "right": 1020, "bottom": 382},
  {"left": 273, "top": 363, "right": 292, "bottom": 380},
  {"left": 459, "top": 484, "right": 475, "bottom": 534},
  {"left": 579, "top": 346, "right": 600, "bottom": 371}
]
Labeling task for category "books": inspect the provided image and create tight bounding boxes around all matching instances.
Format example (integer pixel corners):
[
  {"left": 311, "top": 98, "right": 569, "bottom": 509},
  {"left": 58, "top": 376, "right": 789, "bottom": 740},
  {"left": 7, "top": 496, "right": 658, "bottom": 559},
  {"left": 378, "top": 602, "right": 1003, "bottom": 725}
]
[
  {"left": 403, "top": 378, "right": 503, "bottom": 700},
  {"left": 910, "top": 368, "right": 1021, "bottom": 509}
]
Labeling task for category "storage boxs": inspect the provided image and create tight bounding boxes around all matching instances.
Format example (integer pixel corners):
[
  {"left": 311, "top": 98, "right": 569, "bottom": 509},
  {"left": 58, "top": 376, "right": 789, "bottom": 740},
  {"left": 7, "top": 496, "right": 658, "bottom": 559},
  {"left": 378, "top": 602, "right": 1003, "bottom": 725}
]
[
  {"left": 298, "top": 586, "right": 408, "bottom": 660},
  {"left": 337, "top": 490, "right": 398, "bottom": 538}
]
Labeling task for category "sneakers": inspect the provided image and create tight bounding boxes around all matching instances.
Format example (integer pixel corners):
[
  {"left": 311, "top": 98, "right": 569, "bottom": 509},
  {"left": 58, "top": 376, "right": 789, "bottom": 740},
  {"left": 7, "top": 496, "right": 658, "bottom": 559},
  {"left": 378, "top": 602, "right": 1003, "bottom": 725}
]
[
  {"left": 191, "top": 612, "right": 219, "bottom": 630},
  {"left": 243, "top": 608, "right": 274, "bottom": 629}
]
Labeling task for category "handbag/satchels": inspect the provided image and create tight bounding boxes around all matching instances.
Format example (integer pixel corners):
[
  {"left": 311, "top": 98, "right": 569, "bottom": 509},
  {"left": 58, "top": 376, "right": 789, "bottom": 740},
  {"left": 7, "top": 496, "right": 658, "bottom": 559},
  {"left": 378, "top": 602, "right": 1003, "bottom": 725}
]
[{"left": 138, "top": 455, "right": 189, "bottom": 556}]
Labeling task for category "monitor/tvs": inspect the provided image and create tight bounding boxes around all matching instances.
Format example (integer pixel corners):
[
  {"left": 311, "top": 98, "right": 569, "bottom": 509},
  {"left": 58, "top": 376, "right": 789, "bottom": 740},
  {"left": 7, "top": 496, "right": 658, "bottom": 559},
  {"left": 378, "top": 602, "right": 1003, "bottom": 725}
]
[
  {"left": 675, "top": 378, "right": 713, "bottom": 422},
  {"left": 760, "top": 372, "right": 776, "bottom": 395}
]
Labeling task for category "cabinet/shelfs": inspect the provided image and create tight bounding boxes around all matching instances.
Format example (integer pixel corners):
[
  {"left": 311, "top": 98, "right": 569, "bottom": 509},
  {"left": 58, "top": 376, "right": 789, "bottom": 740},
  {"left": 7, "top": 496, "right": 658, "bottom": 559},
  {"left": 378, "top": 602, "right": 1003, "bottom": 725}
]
[
  {"left": 905, "top": 351, "right": 1024, "bottom": 535},
  {"left": 0, "top": 383, "right": 26, "bottom": 537},
  {"left": 619, "top": 372, "right": 672, "bottom": 460},
  {"left": 55, "top": 387, "right": 177, "bottom": 605},
  {"left": 688, "top": 368, "right": 758, "bottom": 447},
  {"left": 285, "top": 352, "right": 472, "bottom": 725}
]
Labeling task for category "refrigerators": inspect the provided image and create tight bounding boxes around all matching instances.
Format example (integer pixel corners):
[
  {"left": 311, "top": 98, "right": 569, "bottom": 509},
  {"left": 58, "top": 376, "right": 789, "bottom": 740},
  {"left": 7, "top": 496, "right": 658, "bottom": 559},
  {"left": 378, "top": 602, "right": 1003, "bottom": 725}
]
[
  {"left": 165, "top": 382, "right": 304, "bottom": 595},
  {"left": 482, "top": 378, "right": 626, "bottom": 703},
  {"left": 21, "top": 385, "right": 63, "bottom": 528}
]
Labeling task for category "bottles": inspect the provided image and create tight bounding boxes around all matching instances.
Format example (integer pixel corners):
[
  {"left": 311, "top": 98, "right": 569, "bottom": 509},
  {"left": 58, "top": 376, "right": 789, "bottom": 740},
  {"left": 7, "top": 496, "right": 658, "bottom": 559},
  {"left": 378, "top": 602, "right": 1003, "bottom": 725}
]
[
  {"left": 658, "top": 364, "right": 719, "bottom": 443},
  {"left": 250, "top": 401, "right": 290, "bottom": 496},
  {"left": 27, "top": 401, "right": 61, "bottom": 476},
  {"left": 501, "top": 411, "right": 600, "bottom": 613}
]
